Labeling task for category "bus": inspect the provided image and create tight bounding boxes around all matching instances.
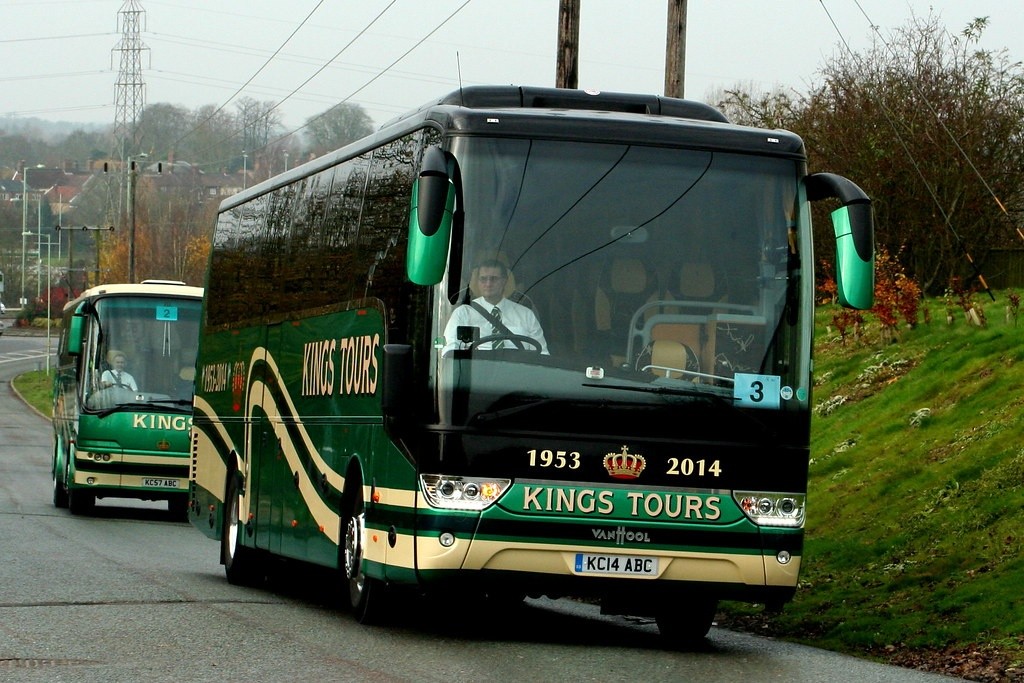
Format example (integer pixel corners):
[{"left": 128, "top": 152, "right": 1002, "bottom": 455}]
[
  {"left": 50, "top": 280, "right": 205, "bottom": 521},
  {"left": 189, "top": 84, "right": 877, "bottom": 645}
]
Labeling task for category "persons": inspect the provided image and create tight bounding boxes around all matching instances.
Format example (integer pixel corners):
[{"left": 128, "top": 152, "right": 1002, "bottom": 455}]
[
  {"left": 442, "top": 259, "right": 549, "bottom": 359},
  {"left": 101, "top": 354, "right": 138, "bottom": 392}
]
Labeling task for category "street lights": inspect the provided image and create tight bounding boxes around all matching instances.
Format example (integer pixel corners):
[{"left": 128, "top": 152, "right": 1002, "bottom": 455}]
[
  {"left": 127, "top": 152, "right": 149, "bottom": 215},
  {"left": 22, "top": 231, "right": 50, "bottom": 377},
  {"left": 59, "top": 204, "right": 78, "bottom": 263},
  {"left": 241, "top": 151, "right": 246, "bottom": 189},
  {"left": 282, "top": 150, "right": 289, "bottom": 170},
  {"left": 10, "top": 198, "right": 41, "bottom": 297}
]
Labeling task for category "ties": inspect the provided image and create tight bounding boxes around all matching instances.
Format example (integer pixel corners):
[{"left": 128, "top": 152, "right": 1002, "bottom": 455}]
[
  {"left": 491, "top": 307, "right": 505, "bottom": 350},
  {"left": 117, "top": 372, "right": 122, "bottom": 387}
]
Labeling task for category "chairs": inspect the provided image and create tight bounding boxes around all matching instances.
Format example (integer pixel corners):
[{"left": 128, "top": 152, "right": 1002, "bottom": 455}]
[{"left": 463, "top": 254, "right": 729, "bottom": 384}]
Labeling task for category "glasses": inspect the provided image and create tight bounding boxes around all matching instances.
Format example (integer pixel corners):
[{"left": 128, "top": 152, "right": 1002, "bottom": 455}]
[{"left": 478, "top": 276, "right": 504, "bottom": 284}]
